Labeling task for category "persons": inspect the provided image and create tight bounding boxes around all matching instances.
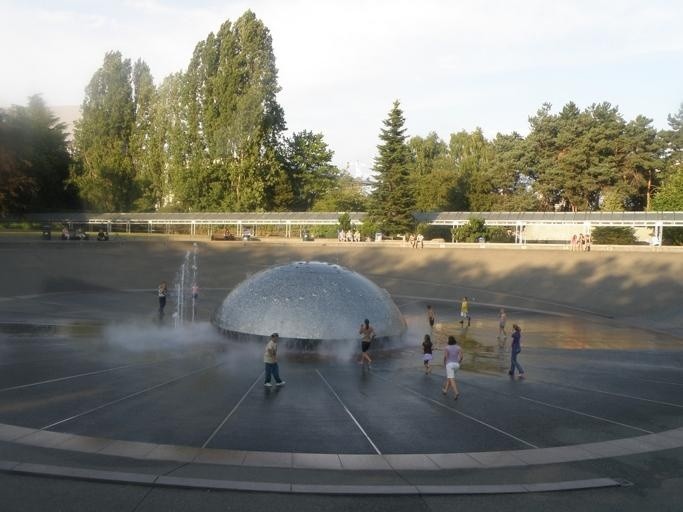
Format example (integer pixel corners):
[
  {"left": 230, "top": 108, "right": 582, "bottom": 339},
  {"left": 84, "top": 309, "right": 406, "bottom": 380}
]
[
  {"left": 191, "top": 282, "right": 199, "bottom": 304},
  {"left": 584, "top": 234, "right": 592, "bottom": 251},
  {"left": 421, "top": 334, "right": 434, "bottom": 374},
  {"left": 427, "top": 304, "right": 434, "bottom": 330},
  {"left": 414, "top": 234, "right": 424, "bottom": 248},
  {"left": 570, "top": 234, "right": 576, "bottom": 251},
  {"left": 408, "top": 233, "right": 416, "bottom": 248},
  {"left": 508, "top": 324, "right": 524, "bottom": 375},
  {"left": 496, "top": 308, "right": 506, "bottom": 338},
  {"left": 650, "top": 234, "right": 658, "bottom": 246},
  {"left": 353, "top": 231, "right": 360, "bottom": 242},
  {"left": 458, "top": 297, "right": 470, "bottom": 323},
  {"left": 263, "top": 333, "right": 285, "bottom": 387},
  {"left": 357, "top": 318, "right": 375, "bottom": 365},
  {"left": 441, "top": 335, "right": 463, "bottom": 400},
  {"left": 156, "top": 281, "right": 168, "bottom": 315},
  {"left": 61, "top": 226, "right": 108, "bottom": 241},
  {"left": 575, "top": 233, "right": 584, "bottom": 251},
  {"left": 338, "top": 229, "right": 345, "bottom": 241},
  {"left": 345, "top": 230, "right": 352, "bottom": 242}
]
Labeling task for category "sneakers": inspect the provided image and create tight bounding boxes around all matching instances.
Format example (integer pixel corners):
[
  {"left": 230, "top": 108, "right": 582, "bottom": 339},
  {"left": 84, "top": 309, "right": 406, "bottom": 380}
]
[
  {"left": 264, "top": 382, "right": 272, "bottom": 387},
  {"left": 441, "top": 387, "right": 460, "bottom": 401},
  {"left": 275, "top": 381, "right": 286, "bottom": 386},
  {"left": 508, "top": 372, "right": 524, "bottom": 377}
]
